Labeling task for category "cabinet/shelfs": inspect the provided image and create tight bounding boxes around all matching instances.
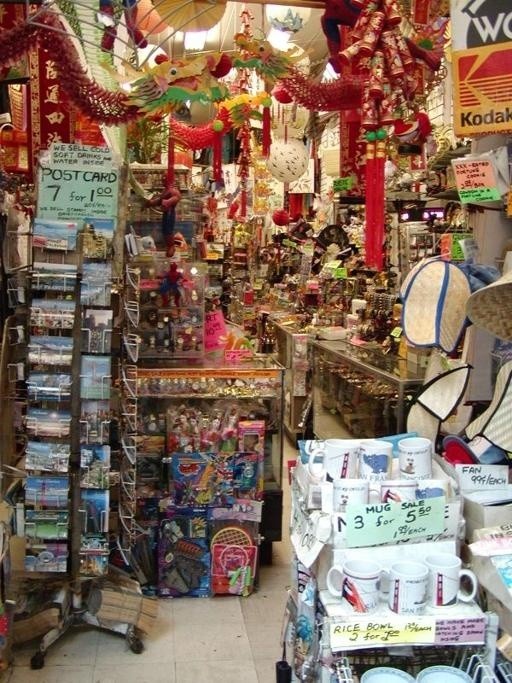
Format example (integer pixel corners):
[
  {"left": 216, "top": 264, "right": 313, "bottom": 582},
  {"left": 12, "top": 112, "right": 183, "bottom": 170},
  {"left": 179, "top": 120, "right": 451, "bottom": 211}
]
[
  {"left": 15, "top": 210, "right": 161, "bottom": 671},
  {"left": 126, "top": 325, "right": 287, "bottom": 583}
]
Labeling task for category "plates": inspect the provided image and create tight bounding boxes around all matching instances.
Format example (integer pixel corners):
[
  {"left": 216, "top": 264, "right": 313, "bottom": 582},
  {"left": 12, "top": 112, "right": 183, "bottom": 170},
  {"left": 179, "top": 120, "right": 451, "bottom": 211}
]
[
  {"left": 415, "top": 664, "right": 475, "bottom": 683},
  {"left": 359, "top": 666, "right": 416, "bottom": 683}
]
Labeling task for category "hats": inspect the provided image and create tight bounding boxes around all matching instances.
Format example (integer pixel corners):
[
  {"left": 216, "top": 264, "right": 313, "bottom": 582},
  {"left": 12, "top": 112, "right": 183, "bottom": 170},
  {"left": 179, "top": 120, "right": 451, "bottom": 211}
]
[
  {"left": 464, "top": 361, "right": 512, "bottom": 452},
  {"left": 399, "top": 258, "right": 471, "bottom": 353},
  {"left": 465, "top": 265, "right": 512, "bottom": 342},
  {"left": 406, "top": 363, "right": 471, "bottom": 450}
]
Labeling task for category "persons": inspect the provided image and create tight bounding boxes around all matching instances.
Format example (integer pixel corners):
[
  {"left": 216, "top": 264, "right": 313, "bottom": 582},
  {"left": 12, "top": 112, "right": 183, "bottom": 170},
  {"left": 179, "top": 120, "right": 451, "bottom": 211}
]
[
  {"left": 155, "top": 259, "right": 190, "bottom": 308},
  {"left": 145, "top": 168, "right": 181, "bottom": 257}
]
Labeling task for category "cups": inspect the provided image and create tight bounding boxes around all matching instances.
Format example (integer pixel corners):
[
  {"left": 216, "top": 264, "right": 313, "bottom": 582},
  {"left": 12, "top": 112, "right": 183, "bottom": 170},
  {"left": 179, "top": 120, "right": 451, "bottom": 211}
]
[
  {"left": 418, "top": 479, "right": 452, "bottom": 501},
  {"left": 325, "top": 558, "right": 384, "bottom": 615},
  {"left": 378, "top": 479, "right": 417, "bottom": 505},
  {"left": 356, "top": 438, "right": 394, "bottom": 484},
  {"left": 379, "top": 560, "right": 430, "bottom": 617},
  {"left": 307, "top": 438, "right": 359, "bottom": 484},
  {"left": 396, "top": 436, "right": 435, "bottom": 482},
  {"left": 421, "top": 551, "right": 479, "bottom": 610},
  {"left": 332, "top": 477, "right": 381, "bottom": 515}
]
